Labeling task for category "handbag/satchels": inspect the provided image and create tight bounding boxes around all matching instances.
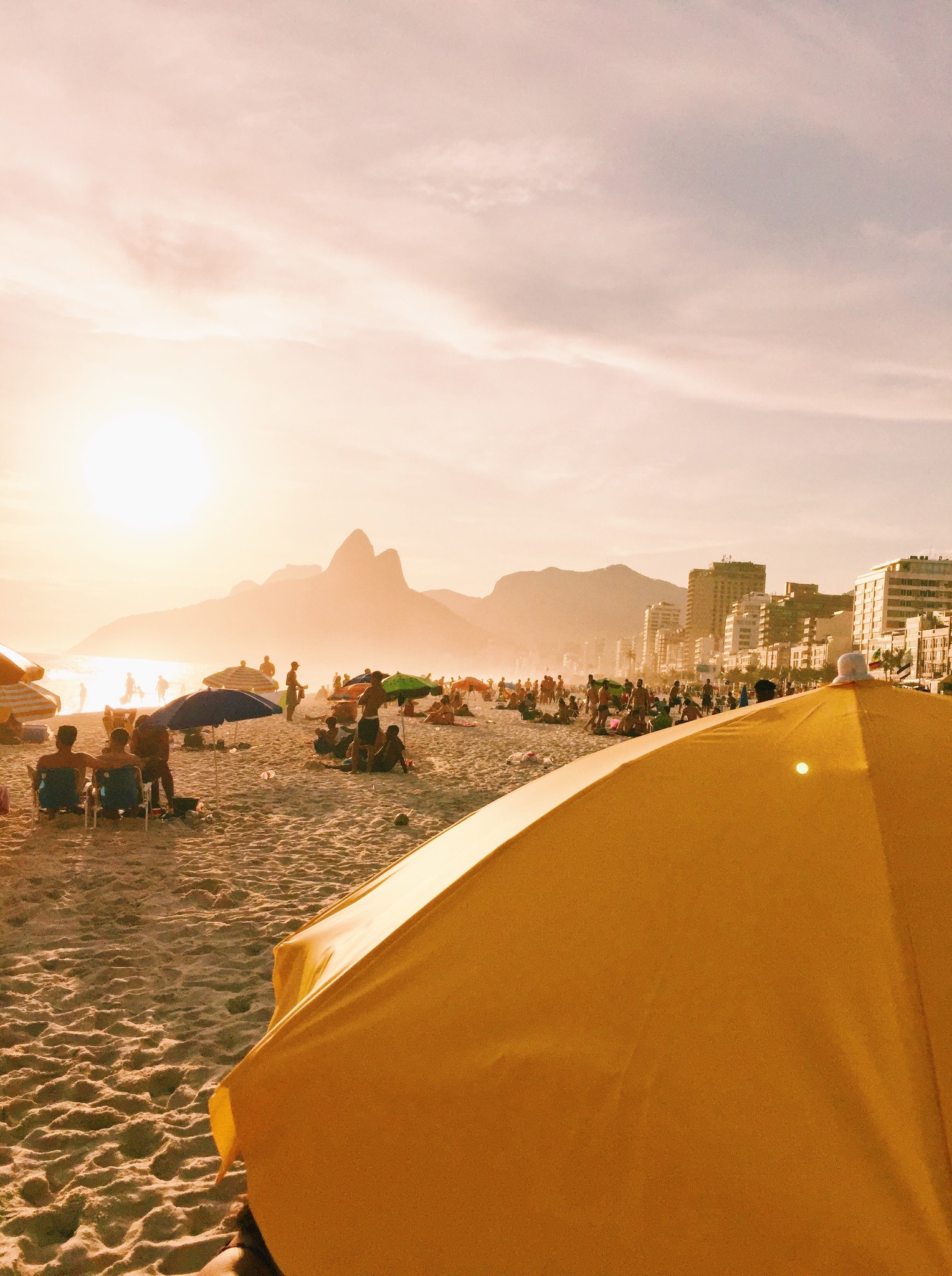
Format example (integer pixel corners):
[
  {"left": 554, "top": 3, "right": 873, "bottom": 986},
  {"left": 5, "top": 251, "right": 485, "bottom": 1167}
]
[
  {"left": 522, "top": 750, "right": 537, "bottom": 762},
  {"left": 505, "top": 751, "right": 522, "bottom": 765},
  {"left": 542, "top": 757, "right": 555, "bottom": 766}
]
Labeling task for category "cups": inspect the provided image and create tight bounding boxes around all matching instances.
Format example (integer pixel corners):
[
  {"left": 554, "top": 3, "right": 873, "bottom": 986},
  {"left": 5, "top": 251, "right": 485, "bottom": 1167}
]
[
  {"left": 152, "top": 808, "right": 160, "bottom": 814},
  {"left": 435, "top": 727, "right": 440, "bottom": 731}
]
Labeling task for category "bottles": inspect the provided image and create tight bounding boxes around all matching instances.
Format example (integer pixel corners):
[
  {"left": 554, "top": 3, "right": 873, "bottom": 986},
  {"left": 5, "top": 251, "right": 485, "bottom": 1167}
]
[
  {"left": 197, "top": 802, "right": 204, "bottom": 811},
  {"left": 258, "top": 769, "right": 275, "bottom": 780}
]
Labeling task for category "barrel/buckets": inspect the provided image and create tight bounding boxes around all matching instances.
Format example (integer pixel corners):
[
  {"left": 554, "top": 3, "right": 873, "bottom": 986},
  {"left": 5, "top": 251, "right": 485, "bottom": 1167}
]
[{"left": 171, "top": 796, "right": 200, "bottom": 816}]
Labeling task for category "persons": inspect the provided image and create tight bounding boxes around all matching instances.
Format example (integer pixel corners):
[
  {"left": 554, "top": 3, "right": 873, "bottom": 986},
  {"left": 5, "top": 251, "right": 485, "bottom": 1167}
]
[
  {"left": 1, "top": 712, "right": 30, "bottom": 744},
  {"left": 94, "top": 728, "right": 144, "bottom": 809},
  {"left": 196, "top": 1190, "right": 284, "bottom": 1276},
  {"left": 240, "top": 659, "right": 247, "bottom": 667},
  {"left": 305, "top": 669, "right": 408, "bottom": 776},
  {"left": 259, "top": 655, "right": 276, "bottom": 678},
  {"left": 417, "top": 671, "right": 831, "bottom": 738},
  {"left": 129, "top": 715, "right": 175, "bottom": 814},
  {"left": 79, "top": 670, "right": 217, "bottom": 751},
  {"left": 285, "top": 660, "right": 304, "bottom": 722},
  {"left": 26, "top": 726, "right": 105, "bottom": 813}
]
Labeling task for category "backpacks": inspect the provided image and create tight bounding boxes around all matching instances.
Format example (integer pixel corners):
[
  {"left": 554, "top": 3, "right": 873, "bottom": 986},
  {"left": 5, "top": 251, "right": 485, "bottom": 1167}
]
[
  {"left": 518, "top": 700, "right": 527, "bottom": 712},
  {"left": 182, "top": 729, "right": 203, "bottom": 749}
]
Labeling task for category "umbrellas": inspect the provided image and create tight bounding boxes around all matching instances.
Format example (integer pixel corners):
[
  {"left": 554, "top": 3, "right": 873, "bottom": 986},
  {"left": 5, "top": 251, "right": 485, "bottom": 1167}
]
[
  {"left": 203, "top": 665, "right": 279, "bottom": 746},
  {"left": 206, "top": 655, "right": 951, "bottom": 1276},
  {"left": 149, "top": 686, "right": 283, "bottom": 816},
  {"left": 0, "top": 643, "right": 62, "bottom": 724},
  {"left": 326, "top": 670, "right": 443, "bottom": 761}
]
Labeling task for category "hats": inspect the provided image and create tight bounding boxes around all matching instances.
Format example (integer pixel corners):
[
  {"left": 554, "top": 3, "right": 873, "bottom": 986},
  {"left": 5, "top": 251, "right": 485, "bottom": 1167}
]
[{"left": 291, "top": 662, "right": 299, "bottom": 666}]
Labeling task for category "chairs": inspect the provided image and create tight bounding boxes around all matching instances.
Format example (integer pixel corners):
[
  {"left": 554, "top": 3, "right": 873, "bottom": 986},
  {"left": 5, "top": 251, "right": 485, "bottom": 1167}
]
[
  {"left": 93, "top": 765, "right": 156, "bottom": 832},
  {"left": 31, "top": 767, "right": 94, "bottom": 829}
]
